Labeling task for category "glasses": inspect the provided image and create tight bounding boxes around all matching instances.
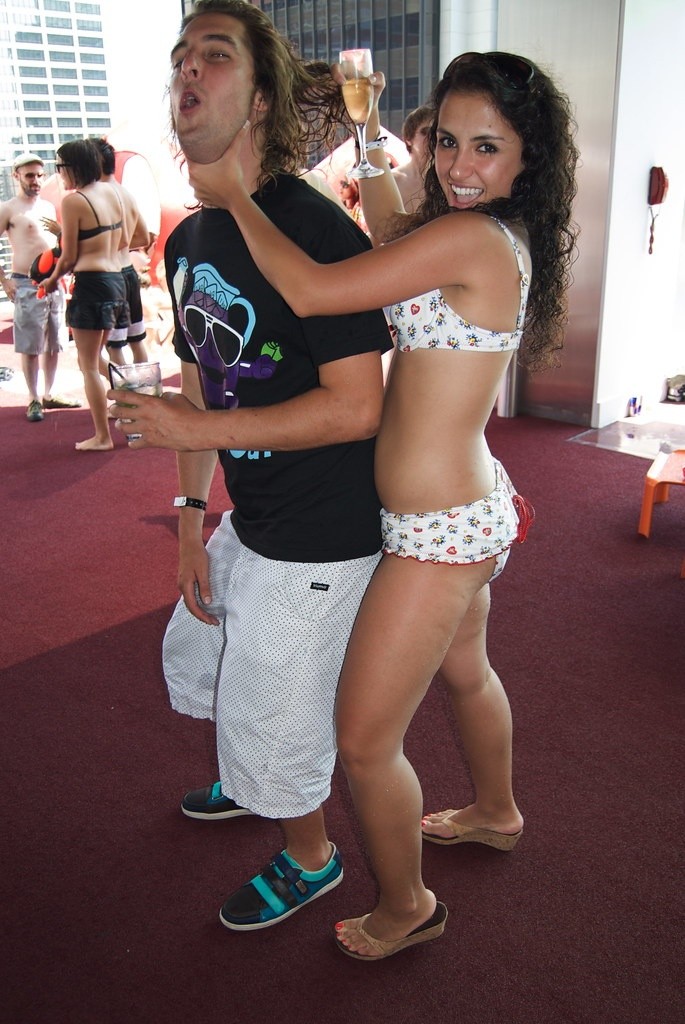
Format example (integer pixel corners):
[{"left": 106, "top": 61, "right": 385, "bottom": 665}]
[
  {"left": 53, "top": 163, "right": 68, "bottom": 172},
  {"left": 25, "top": 172, "right": 45, "bottom": 180},
  {"left": 414, "top": 127, "right": 431, "bottom": 136},
  {"left": 443, "top": 52, "right": 539, "bottom": 97},
  {"left": 340, "top": 181, "right": 350, "bottom": 189}
]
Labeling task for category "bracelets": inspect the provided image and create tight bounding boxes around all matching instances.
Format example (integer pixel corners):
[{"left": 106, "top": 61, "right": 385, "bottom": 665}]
[
  {"left": 174, "top": 496, "right": 208, "bottom": 511},
  {"left": 354, "top": 131, "right": 387, "bottom": 153}
]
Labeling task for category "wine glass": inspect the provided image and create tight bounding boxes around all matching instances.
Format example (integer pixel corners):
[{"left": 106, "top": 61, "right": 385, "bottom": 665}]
[{"left": 336, "top": 48, "right": 385, "bottom": 179}]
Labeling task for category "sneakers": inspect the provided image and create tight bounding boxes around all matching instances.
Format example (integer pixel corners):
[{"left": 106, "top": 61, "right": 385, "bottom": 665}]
[
  {"left": 219, "top": 841, "right": 343, "bottom": 930},
  {"left": 27, "top": 401, "right": 43, "bottom": 420},
  {"left": 180, "top": 782, "right": 257, "bottom": 819}
]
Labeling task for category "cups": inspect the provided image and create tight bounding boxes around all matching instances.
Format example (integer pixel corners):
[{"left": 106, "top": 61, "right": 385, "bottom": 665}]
[{"left": 110, "top": 361, "right": 163, "bottom": 441}]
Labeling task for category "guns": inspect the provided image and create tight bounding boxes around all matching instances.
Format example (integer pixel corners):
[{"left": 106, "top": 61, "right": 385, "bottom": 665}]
[{"left": 28, "top": 245, "right": 62, "bottom": 301}]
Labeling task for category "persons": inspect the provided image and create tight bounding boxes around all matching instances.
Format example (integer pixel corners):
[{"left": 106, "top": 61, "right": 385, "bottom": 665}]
[
  {"left": 107, "top": 0, "right": 394, "bottom": 929},
  {"left": 0, "top": 137, "right": 159, "bottom": 451},
  {"left": 340, "top": 176, "right": 368, "bottom": 237},
  {"left": 295, "top": 102, "right": 434, "bottom": 248},
  {"left": 188, "top": 50, "right": 576, "bottom": 961}
]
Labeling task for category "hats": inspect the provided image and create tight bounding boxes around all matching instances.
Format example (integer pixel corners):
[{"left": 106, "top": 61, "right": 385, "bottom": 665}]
[{"left": 14, "top": 153, "right": 42, "bottom": 171}]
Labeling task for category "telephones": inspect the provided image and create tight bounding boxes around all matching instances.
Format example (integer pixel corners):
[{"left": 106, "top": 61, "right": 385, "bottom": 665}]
[{"left": 648, "top": 166, "right": 669, "bottom": 204}]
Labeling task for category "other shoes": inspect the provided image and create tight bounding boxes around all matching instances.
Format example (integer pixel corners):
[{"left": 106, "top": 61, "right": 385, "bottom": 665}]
[{"left": 42, "top": 396, "right": 81, "bottom": 409}]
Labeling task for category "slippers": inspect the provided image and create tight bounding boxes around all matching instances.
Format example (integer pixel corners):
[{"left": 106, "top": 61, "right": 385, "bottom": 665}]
[
  {"left": 422, "top": 809, "right": 523, "bottom": 851},
  {"left": 335, "top": 901, "right": 448, "bottom": 961}
]
